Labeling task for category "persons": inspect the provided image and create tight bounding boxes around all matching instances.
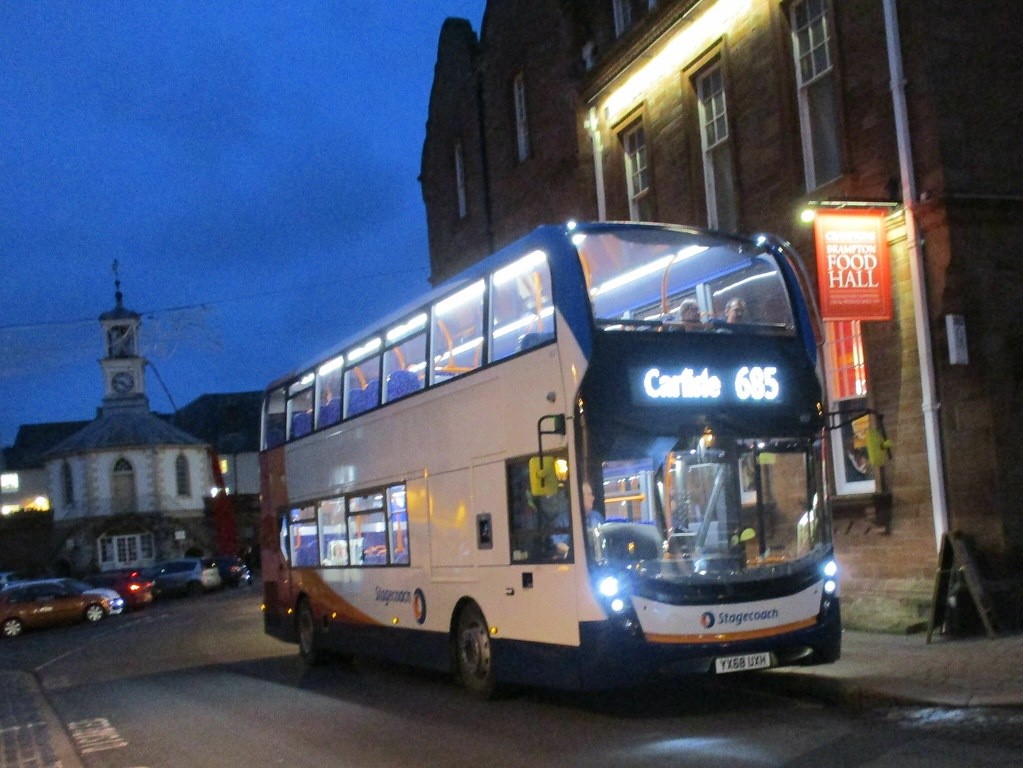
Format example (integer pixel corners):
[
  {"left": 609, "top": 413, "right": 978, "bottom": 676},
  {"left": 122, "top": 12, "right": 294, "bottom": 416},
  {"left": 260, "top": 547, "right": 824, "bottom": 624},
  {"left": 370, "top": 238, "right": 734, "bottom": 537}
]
[
  {"left": 715, "top": 297, "right": 753, "bottom": 335},
  {"left": 670, "top": 298, "right": 711, "bottom": 334}
]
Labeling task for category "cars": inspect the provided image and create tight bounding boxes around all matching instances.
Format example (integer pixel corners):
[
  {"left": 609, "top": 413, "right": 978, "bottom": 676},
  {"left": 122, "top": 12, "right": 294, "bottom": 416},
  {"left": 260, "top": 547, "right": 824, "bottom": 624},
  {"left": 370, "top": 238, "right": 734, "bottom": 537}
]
[{"left": 0, "top": 557, "right": 245, "bottom": 638}]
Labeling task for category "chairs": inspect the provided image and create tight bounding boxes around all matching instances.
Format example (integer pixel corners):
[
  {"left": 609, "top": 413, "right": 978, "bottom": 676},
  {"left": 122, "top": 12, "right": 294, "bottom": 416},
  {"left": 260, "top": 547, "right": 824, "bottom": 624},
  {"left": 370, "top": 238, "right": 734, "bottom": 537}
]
[{"left": 267, "top": 302, "right": 736, "bottom": 589}]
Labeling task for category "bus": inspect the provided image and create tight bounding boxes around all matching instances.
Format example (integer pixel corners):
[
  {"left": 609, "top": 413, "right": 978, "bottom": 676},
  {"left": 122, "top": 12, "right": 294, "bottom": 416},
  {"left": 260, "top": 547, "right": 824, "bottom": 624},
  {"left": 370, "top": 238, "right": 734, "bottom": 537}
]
[{"left": 252, "top": 220, "right": 844, "bottom": 700}]
